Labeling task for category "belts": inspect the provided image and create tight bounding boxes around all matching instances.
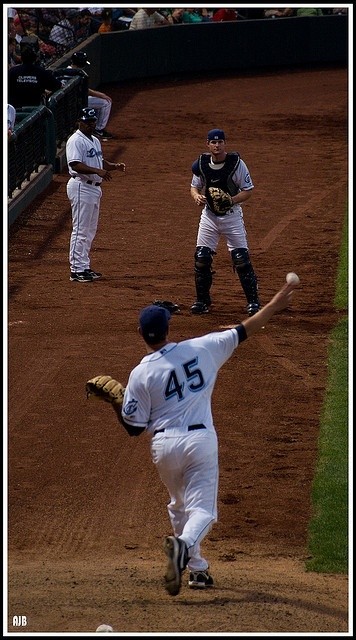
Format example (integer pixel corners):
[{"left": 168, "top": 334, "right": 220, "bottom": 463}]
[
  {"left": 153, "top": 423, "right": 206, "bottom": 434},
  {"left": 72, "top": 175, "right": 101, "bottom": 186}
]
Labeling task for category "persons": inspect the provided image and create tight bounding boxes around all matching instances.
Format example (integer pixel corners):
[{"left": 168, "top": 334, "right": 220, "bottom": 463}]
[
  {"left": 8, "top": 17, "right": 22, "bottom": 44},
  {"left": 190, "top": 129, "right": 262, "bottom": 316},
  {"left": 61, "top": 51, "right": 114, "bottom": 140},
  {"left": 65, "top": 107, "right": 125, "bottom": 283},
  {"left": 129, "top": 8, "right": 168, "bottom": 30},
  {"left": 18, "top": 13, "right": 58, "bottom": 60},
  {"left": 7, "top": 104, "right": 16, "bottom": 136},
  {"left": 8, "top": 35, "right": 65, "bottom": 107},
  {"left": 97, "top": 10, "right": 113, "bottom": 33},
  {"left": 113, "top": 8, "right": 137, "bottom": 28},
  {"left": 8, "top": 36, "right": 22, "bottom": 70},
  {"left": 169, "top": 8, "right": 348, "bottom": 25},
  {"left": 77, "top": 9, "right": 92, "bottom": 43},
  {"left": 49, "top": 8, "right": 80, "bottom": 50},
  {"left": 85, "top": 281, "right": 295, "bottom": 596}
]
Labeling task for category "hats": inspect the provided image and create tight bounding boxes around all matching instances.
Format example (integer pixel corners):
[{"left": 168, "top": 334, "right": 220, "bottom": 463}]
[
  {"left": 66, "top": 9, "right": 77, "bottom": 19},
  {"left": 139, "top": 305, "right": 171, "bottom": 337},
  {"left": 208, "top": 129, "right": 225, "bottom": 140}
]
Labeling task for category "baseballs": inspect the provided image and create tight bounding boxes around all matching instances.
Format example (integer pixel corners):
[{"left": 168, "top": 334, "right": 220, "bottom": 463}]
[{"left": 286, "top": 272, "right": 299, "bottom": 286}]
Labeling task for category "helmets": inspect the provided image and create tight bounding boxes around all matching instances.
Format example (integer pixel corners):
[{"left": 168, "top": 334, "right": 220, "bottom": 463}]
[
  {"left": 78, "top": 107, "right": 99, "bottom": 120},
  {"left": 71, "top": 51, "right": 90, "bottom": 68}
]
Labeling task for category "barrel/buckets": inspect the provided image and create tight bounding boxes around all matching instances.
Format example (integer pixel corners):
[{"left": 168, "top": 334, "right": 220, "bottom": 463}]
[{"left": 88, "top": 96, "right": 112, "bottom": 131}]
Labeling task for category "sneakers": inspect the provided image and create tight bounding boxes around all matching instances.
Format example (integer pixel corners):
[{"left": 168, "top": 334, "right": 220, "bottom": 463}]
[
  {"left": 188, "top": 570, "right": 214, "bottom": 589},
  {"left": 248, "top": 303, "right": 261, "bottom": 316},
  {"left": 162, "top": 536, "right": 191, "bottom": 595},
  {"left": 69, "top": 271, "right": 93, "bottom": 282},
  {"left": 191, "top": 301, "right": 209, "bottom": 313},
  {"left": 92, "top": 129, "right": 113, "bottom": 139},
  {"left": 84, "top": 269, "right": 101, "bottom": 279}
]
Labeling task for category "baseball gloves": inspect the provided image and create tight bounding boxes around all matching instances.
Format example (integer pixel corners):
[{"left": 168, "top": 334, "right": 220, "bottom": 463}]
[
  {"left": 86, "top": 376, "right": 125, "bottom": 415},
  {"left": 204, "top": 186, "right": 233, "bottom": 216}
]
